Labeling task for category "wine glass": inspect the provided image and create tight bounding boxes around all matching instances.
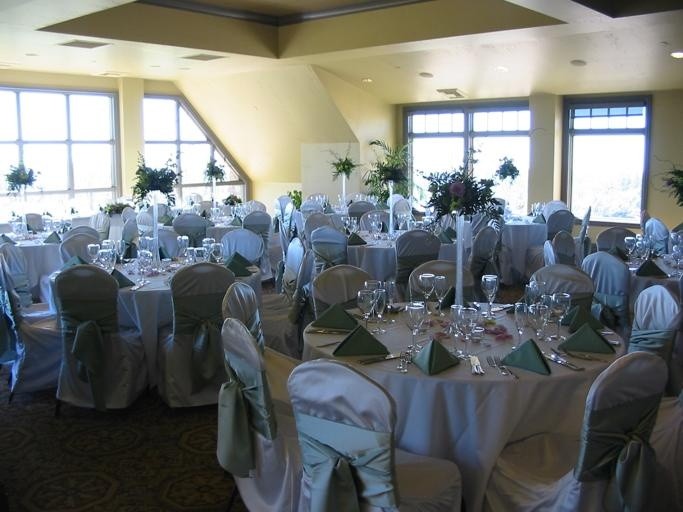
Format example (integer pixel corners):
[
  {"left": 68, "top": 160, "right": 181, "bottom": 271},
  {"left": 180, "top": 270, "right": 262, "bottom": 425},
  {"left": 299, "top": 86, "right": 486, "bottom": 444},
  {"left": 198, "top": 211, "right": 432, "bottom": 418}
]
[
  {"left": 625, "top": 233, "right": 683, "bottom": 277},
  {"left": 504, "top": 201, "right": 545, "bottom": 222},
  {"left": 87, "top": 236, "right": 223, "bottom": 284},
  {"left": 310, "top": 194, "right": 378, "bottom": 212},
  {"left": 357, "top": 273, "right": 570, "bottom": 361},
  {"left": 341, "top": 214, "right": 434, "bottom": 246},
  {"left": 194, "top": 203, "right": 252, "bottom": 224},
  {"left": 13, "top": 217, "right": 74, "bottom": 241}
]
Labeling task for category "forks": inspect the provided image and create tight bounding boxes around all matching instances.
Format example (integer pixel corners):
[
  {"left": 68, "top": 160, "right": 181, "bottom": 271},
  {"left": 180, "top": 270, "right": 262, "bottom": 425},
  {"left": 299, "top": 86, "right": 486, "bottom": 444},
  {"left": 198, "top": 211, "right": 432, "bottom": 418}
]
[
  {"left": 550, "top": 347, "right": 592, "bottom": 358},
  {"left": 486, "top": 355, "right": 519, "bottom": 379}
]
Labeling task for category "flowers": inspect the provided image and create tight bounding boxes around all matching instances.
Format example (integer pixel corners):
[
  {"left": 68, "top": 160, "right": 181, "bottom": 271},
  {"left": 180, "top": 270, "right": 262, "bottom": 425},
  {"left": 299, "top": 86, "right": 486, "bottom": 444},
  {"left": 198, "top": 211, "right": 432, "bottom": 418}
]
[{"left": 420, "top": 166, "right": 503, "bottom": 222}]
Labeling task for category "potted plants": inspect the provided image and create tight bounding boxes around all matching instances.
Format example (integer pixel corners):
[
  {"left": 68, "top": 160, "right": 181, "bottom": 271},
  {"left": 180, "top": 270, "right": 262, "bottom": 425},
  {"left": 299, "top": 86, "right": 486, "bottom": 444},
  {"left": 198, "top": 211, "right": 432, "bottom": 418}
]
[
  {"left": 132, "top": 151, "right": 187, "bottom": 272},
  {"left": 328, "top": 141, "right": 366, "bottom": 204},
  {"left": 204, "top": 158, "right": 226, "bottom": 210},
  {"left": 495, "top": 157, "right": 519, "bottom": 215},
  {"left": 362, "top": 139, "right": 413, "bottom": 234},
  {"left": 5, "top": 161, "right": 41, "bottom": 233}
]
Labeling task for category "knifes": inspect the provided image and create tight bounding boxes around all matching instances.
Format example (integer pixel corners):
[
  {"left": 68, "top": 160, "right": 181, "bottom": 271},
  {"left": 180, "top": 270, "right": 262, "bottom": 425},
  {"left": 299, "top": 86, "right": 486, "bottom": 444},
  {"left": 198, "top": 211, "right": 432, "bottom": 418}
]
[
  {"left": 305, "top": 329, "right": 350, "bottom": 334},
  {"left": 359, "top": 353, "right": 400, "bottom": 365},
  {"left": 470, "top": 356, "right": 485, "bottom": 375},
  {"left": 542, "top": 351, "right": 584, "bottom": 371}
]
